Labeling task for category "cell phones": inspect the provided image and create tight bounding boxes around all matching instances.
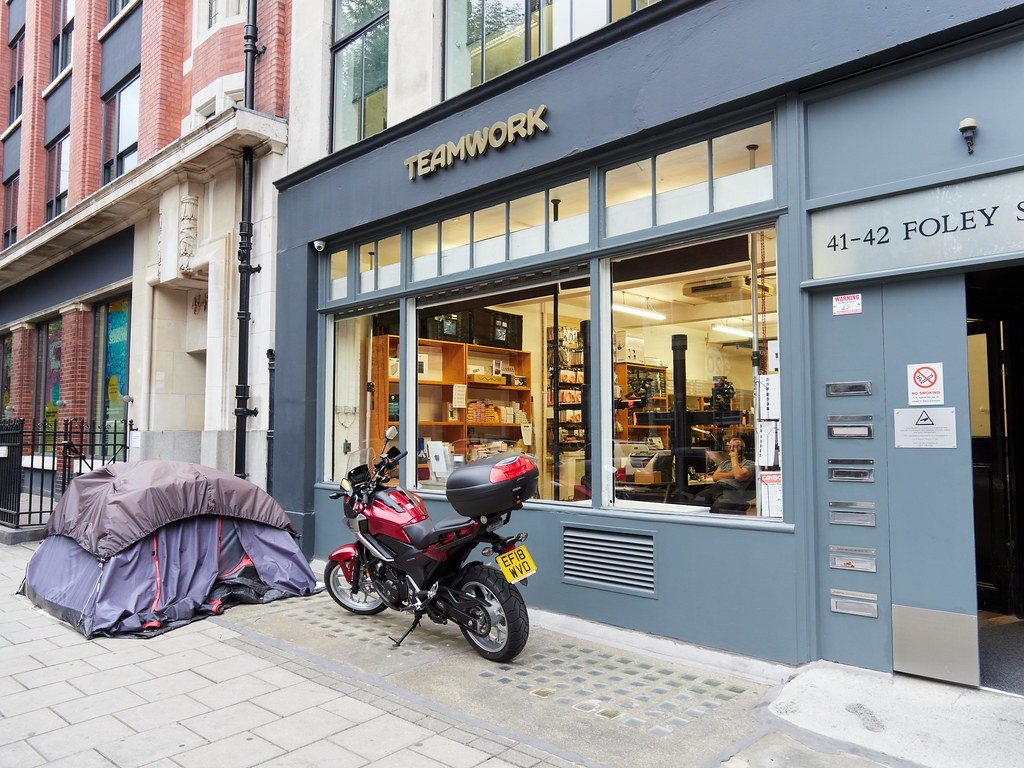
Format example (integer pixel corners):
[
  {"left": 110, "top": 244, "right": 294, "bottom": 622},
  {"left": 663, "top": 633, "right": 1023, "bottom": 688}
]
[{"left": 738, "top": 446, "right": 745, "bottom": 456}]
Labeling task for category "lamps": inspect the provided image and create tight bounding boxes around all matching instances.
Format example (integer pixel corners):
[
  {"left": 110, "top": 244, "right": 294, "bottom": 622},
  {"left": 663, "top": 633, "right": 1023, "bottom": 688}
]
[
  {"left": 711, "top": 317, "right": 754, "bottom": 337},
  {"left": 612, "top": 291, "right": 666, "bottom": 321}
]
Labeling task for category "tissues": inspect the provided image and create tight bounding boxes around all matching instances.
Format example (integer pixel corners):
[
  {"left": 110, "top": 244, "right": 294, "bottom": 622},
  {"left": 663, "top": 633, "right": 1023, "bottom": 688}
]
[{"left": 634, "top": 453, "right": 662, "bottom": 484}]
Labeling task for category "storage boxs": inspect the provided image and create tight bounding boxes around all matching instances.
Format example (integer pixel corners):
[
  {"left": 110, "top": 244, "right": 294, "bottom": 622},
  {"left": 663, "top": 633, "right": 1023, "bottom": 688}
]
[
  {"left": 421, "top": 310, "right": 470, "bottom": 343},
  {"left": 615, "top": 329, "right": 645, "bottom": 364},
  {"left": 388, "top": 353, "right": 529, "bottom": 454},
  {"left": 470, "top": 307, "right": 523, "bottom": 351}
]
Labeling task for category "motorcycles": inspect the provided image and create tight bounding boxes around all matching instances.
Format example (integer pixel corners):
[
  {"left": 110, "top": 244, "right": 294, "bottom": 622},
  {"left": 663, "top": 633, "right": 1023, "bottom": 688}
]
[{"left": 323, "top": 424, "right": 539, "bottom": 663}]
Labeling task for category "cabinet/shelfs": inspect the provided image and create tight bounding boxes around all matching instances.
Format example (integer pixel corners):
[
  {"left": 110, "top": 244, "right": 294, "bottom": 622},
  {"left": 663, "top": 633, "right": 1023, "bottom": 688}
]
[
  {"left": 368, "top": 334, "right": 534, "bottom": 480},
  {"left": 614, "top": 363, "right": 671, "bottom": 450},
  {"left": 669, "top": 392, "right": 734, "bottom": 441}
]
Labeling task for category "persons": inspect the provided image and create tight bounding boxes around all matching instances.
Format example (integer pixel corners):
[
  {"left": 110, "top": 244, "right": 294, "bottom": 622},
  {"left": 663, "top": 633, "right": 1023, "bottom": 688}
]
[{"left": 692, "top": 437, "right": 756, "bottom": 513}]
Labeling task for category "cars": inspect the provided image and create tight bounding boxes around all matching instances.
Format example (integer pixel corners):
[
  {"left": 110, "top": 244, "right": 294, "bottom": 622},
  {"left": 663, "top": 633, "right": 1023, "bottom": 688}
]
[{"left": 581, "top": 438, "right": 654, "bottom": 468}]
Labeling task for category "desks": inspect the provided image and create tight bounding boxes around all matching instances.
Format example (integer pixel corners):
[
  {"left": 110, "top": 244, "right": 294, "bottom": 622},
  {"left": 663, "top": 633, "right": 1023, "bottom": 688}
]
[{"left": 615, "top": 479, "right": 716, "bottom": 491}]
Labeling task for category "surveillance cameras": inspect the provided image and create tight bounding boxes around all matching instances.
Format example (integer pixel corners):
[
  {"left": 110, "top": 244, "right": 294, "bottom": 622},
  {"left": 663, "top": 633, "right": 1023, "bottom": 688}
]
[{"left": 313, "top": 241, "right": 326, "bottom": 251}]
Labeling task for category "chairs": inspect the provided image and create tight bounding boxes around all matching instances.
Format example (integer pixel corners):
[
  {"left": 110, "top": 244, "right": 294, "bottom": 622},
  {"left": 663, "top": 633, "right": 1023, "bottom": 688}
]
[{"left": 711, "top": 480, "right": 756, "bottom": 515}]
